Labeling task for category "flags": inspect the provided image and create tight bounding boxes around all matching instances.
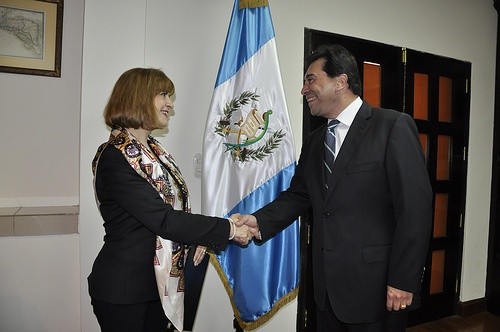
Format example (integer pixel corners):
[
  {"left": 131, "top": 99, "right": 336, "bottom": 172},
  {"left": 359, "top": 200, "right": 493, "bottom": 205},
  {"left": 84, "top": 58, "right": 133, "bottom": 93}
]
[{"left": 200, "top": 0, "right": 299, "bottom": 332}]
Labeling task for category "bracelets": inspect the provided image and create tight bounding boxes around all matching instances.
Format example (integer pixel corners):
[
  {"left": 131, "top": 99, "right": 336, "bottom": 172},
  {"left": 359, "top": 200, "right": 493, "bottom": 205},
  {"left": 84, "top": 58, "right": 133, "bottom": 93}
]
[{"left": 226, "top": 218, "right": 235, "bottom": 240}]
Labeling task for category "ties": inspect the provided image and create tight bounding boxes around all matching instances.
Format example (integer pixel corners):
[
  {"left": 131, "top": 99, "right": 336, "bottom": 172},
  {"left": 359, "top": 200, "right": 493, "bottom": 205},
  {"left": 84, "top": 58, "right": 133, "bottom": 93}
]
[{"left": 323, "top": 120, "right": 341, "bottom": 194}]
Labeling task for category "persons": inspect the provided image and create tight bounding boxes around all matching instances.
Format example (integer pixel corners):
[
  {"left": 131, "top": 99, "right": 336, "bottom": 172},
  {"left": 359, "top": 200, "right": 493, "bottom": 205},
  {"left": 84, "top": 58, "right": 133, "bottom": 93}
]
[
  {"left": 88, "top": 68, "right": 258, "bottom": 332},
  {"left": 235, "top": 43, "right": 433, "bottom": 332}
]
